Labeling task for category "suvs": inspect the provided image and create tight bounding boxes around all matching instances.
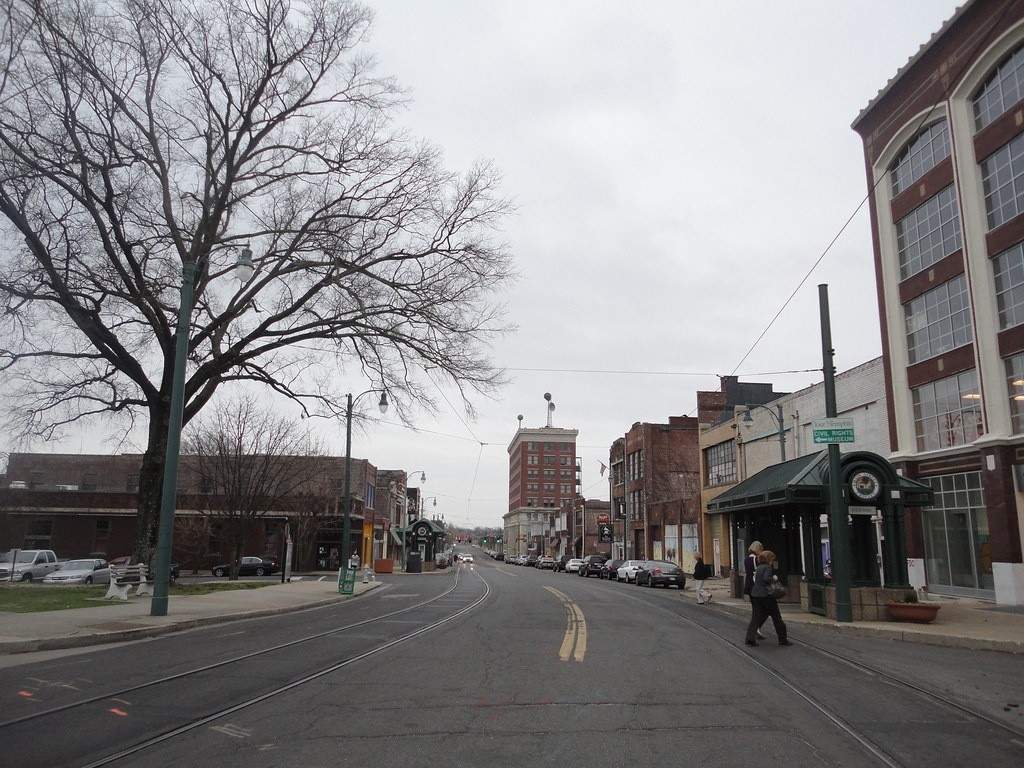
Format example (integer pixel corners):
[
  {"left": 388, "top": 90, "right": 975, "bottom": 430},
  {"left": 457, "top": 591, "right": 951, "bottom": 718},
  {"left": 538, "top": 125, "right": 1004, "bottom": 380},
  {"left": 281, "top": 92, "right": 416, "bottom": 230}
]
[
  {"left": 553, "top": 554, "right": 575, "bottom": 572},
  {"left": 578, "top": 555, "right": 608, "bottom": 577}
]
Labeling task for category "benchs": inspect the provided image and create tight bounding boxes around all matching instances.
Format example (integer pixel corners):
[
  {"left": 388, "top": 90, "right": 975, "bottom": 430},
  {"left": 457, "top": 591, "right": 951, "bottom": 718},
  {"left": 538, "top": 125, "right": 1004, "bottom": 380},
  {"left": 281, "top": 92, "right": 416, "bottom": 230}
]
[{"left": 104, "top": 563, "right": 155, "bottom": 601}]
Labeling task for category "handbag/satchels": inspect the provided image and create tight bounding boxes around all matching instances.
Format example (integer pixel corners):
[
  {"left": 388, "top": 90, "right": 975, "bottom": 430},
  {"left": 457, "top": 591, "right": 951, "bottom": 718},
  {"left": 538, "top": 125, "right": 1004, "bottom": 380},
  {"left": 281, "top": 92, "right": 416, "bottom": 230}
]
[{"left": 766, "top": 581, "right": 785, "bottom": 598}]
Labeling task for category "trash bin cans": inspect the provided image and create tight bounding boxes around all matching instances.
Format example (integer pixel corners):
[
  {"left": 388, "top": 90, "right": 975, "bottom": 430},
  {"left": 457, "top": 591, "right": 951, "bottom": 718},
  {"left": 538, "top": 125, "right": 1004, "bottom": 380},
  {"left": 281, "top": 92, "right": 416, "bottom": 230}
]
[{"left": 406, "top": 551, "right": 422, "bottom": 573}]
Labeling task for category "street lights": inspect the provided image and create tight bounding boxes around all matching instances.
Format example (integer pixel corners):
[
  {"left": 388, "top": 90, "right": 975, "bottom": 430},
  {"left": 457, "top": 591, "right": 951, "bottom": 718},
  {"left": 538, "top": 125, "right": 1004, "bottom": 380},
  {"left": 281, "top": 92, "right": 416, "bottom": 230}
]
[
  {"left": 342, "top": 388, "right": 390, "bottom": 570},
  {"left": 149, "top": 236, "right": 255, "bottom": 617},
  {"left": 421, "top": 496, "right": 438, "bottom": 518},
  {"left": 743, "top": 403, "right": 786, "bottom": 463},
  {"left": 402, "top": 470, "right": 426, "bottom": 573},
  {"left": 432, "top": 513, "right": 445, "bottom": 523}
]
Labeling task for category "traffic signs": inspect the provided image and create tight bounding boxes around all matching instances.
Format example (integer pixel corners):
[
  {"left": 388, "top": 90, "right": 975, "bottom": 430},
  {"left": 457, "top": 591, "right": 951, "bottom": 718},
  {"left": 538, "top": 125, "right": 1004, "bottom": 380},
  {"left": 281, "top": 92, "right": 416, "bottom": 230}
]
[{"left": 812, "top": 417, "right": 855, "bottom": 444}]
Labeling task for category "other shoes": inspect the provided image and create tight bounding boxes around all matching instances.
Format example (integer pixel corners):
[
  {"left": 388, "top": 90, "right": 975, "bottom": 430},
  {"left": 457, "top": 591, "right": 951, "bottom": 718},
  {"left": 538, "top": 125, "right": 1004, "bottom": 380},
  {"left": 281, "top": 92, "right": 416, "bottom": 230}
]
[
  {"left": 708, "top": 595, "right": 712, "bottom": 601},
  {"left": 698, "top": 603, "right": 704, "bottom": 604},
  {"left": 745, "top": 639, "right": 759, "bottom": 646},
  {"left": 779, "top": 639, "right": 793, "bottom": 646},
  {"left": 756, "top": 628, "right": 764, "bottom": 639}
]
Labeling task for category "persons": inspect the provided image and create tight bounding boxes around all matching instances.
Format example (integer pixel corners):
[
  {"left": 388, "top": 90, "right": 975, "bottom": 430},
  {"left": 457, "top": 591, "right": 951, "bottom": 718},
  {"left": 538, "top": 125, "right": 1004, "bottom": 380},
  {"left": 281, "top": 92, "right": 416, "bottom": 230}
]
[
  {"left": 691, "top": 552, "right": 713, "bottom": 605},
  {"left": 351, "top": 551, "right": 360, "bottom": 569},
  {"left": 744, "top": 551, "right": 794, "bottom": 646},
  {"left": 744, "top": 542, "right": 771, "bottom": 639}
]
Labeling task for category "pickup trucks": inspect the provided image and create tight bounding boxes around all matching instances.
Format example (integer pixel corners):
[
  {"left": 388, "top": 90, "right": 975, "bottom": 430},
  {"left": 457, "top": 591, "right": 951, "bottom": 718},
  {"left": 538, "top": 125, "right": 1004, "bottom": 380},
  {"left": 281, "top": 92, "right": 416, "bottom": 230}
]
[
  {"left": 0, "top": 550, "right": 69, "bottom": 583},
  {"left": 108, "top": 555, "right": 180, "bottom": 585}
]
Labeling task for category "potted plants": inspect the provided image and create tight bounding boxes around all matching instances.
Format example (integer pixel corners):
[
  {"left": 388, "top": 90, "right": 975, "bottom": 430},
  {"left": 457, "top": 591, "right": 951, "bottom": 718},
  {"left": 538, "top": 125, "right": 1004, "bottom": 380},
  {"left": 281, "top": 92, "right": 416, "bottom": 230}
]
[
  {"left": 439, "top": 555, "right": 447, "bottom": 569},
  {"left": 884, "top": 591, "right": 941, "bottom": 624}
]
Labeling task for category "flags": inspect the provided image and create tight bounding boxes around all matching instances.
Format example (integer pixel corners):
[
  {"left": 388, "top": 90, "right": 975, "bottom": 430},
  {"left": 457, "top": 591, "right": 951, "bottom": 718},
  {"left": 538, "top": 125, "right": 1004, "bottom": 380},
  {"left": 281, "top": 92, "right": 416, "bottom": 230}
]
[{"left": 600, "top": 464, "right": 607, "bottom": 476}]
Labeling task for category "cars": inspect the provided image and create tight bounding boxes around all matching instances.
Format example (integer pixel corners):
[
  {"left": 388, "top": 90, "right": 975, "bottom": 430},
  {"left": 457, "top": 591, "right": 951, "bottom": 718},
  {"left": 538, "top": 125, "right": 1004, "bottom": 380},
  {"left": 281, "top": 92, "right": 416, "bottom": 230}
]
[
  {"left": 443, "top": 543, "right": 457, "bottom": 561},
  {"left": 457, "top": 553, "right": 464, "bottom": 560},
  {"left": 211, "top": 556, "right": 279, "bottom": 578},
  {"left": 484, "top": 548, "right": 504, "bottom": 561},
  {"left": 42, "top": 559, "right": 116, "bottom": 585},
  {"left": 616, "top": 561, "right": 645, "bottom": 584},
  {"left": 505, "top": 555, "right": 555, "bottom": 570},
  {"left": 599, "top": 560, "right": 632, "bottom": 580},
  {"left": 565, "top": 559, "right": 585, "bottom": 574},
  {"left": 634, "top": 560, "right": 686, "bottom": 589},
  {"left": 462, "top": 553, "right": 473, "bottom": 563}
]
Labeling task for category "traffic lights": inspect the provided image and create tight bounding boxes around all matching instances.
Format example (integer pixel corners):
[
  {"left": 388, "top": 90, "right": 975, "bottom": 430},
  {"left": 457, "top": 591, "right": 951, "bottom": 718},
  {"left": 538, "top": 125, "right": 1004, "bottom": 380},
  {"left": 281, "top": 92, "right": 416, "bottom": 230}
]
[
  {"left": 484, "top": 537, "right": 486, "bottom": 543},
  {"left": 458, "top": 537, "right": 461, "bottom": 543},
  {"left": 497, "top": 537, "right": 499, "bottom": 542},
  {"left": 469, "top": 537, "right": 471, "bottom": 543}
]
